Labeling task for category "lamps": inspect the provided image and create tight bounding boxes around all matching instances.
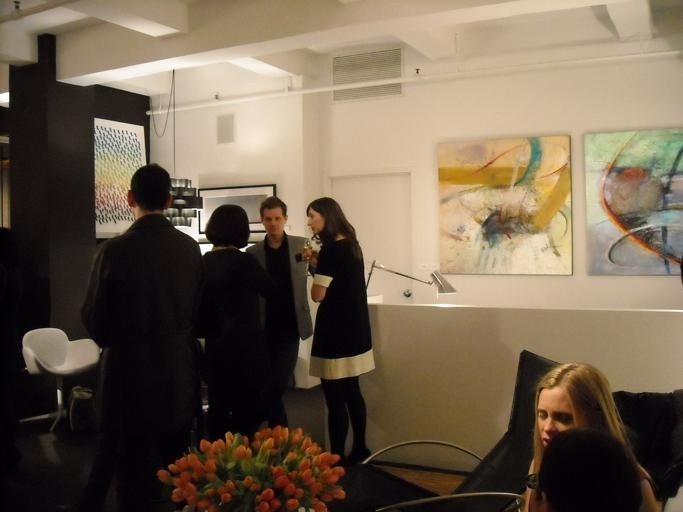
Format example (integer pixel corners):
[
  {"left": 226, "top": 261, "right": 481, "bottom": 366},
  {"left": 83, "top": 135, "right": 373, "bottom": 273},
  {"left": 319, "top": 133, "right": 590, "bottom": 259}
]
[
  {"left": 159, "top": 69, "right": 203, "bottom": 228},
  {"left": 365, "top": 257, "right": 457, "bottom": 298}
]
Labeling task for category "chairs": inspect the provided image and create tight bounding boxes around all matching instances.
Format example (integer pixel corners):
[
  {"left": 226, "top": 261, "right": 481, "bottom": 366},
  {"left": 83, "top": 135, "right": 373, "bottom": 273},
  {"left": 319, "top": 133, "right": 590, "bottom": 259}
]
[
  {"left": 20, "top": 328, "right": 102, "bottom": 434},
  {"left": 360, "top": 349, "right": 681, "bottom": 512}
]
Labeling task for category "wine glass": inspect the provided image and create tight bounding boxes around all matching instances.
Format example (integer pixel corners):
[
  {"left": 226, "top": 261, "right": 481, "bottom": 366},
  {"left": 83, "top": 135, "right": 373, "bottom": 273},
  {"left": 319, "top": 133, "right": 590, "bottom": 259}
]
[{"left": 301, "top": 239, "right": 313, "bottom": 277}]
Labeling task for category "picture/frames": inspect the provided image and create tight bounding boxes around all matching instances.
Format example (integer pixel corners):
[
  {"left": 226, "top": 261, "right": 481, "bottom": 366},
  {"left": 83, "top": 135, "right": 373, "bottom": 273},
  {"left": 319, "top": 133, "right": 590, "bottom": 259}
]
[{"left": 197, "top": 183, "right": 276, "bottom": 234}]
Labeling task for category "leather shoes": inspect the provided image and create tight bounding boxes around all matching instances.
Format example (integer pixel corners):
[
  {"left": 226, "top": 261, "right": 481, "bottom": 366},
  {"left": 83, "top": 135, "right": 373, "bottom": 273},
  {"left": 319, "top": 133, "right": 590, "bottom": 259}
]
[{"left": 349, "top": 449, "right": 371, "bottom": 463}]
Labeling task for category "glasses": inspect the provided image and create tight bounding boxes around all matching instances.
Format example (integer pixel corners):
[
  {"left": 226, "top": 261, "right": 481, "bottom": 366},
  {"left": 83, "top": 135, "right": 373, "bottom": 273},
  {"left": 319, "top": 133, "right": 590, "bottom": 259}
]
[{"left": 522, "top": 473, "right": 538, "bottom": 490}]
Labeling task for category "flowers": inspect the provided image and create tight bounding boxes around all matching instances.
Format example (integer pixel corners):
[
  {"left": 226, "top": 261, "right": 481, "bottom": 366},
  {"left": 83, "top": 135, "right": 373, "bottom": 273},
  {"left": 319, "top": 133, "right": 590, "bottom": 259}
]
[{"left": 154, "top": 422, "right": 348, "bottom": 512}]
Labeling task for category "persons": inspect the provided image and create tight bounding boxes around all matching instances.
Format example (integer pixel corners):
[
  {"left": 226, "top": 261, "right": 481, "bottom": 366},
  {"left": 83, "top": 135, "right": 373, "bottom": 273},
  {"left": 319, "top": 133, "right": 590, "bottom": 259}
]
[
  {"left": 524, "top": 422, "right": 644, "bottom": 512},
  {"left": 244, "top": 195, "right": 313, "bottom": 430},
  {"left": 193, "top": 202, "right": 282, "bottom": 444},
  {"left": 522, "top": 360, "right": 659, "bottom": 512},
  {"left": 302, "top": 195, "right": 375, "bottom": 469},
  {"left": 80, "top": 159, "right": 204, "bottom": 512}
]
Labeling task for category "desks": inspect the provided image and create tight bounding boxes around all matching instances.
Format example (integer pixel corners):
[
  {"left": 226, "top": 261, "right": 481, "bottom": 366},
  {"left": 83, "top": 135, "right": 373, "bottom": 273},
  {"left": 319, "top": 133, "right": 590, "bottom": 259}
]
[{"left": 311, "top": 451, "right": 447, "bottom": 511}]
